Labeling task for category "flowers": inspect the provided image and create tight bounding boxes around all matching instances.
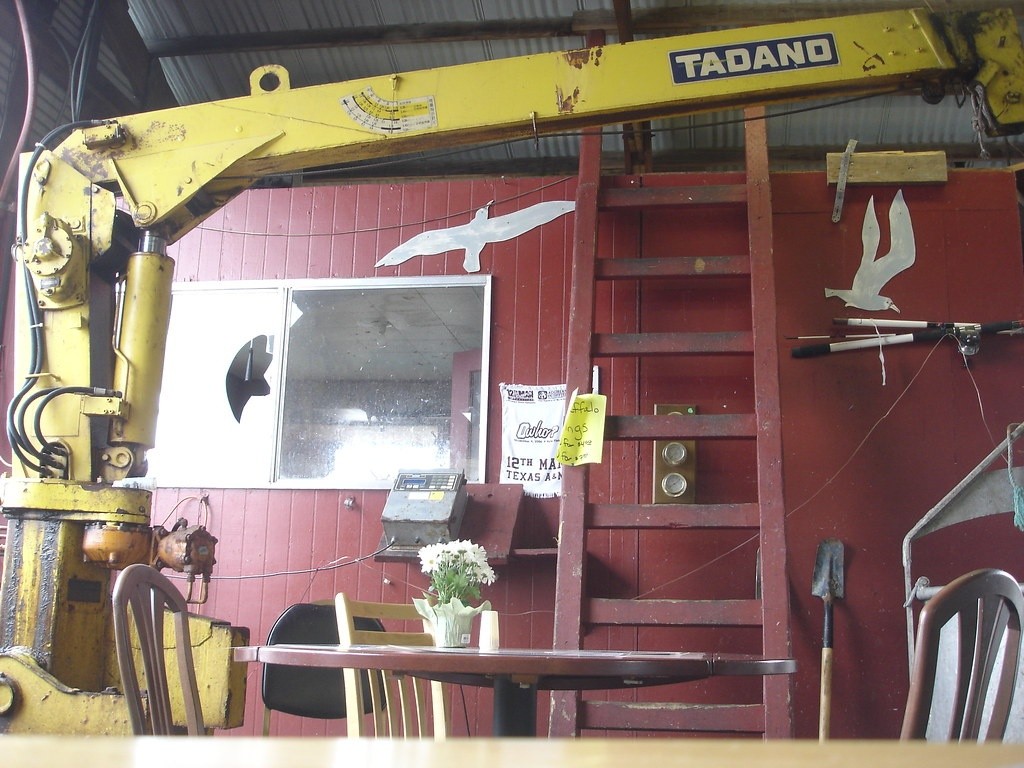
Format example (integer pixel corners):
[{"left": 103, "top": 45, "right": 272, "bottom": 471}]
[{"left": 416, "top": 538, "right": 497, "bottom": 604}]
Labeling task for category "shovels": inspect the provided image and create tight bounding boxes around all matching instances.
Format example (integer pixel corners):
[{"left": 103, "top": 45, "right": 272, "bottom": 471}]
[{"left": 812, "top": 537, "right": 846, "bottom": 743}]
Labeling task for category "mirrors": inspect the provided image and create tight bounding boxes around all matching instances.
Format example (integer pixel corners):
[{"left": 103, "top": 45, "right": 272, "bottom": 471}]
[{"left": 266, "top": 272, "right": 492, "bottom": 489}]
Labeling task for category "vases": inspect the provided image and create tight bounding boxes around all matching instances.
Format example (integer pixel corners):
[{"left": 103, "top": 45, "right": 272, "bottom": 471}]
[{"left": 410, "top": 597, "right": 492, "bottom": 649}]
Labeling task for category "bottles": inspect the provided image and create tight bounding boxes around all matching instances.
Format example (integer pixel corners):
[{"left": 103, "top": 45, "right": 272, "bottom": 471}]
[{"left": 479, "top": 608, "right": 500, "bottom": 653}]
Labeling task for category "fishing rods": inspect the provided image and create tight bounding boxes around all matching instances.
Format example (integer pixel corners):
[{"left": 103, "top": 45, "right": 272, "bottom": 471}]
[{"left": 783, "top": 316, "right": 1024, "bottom": 359}]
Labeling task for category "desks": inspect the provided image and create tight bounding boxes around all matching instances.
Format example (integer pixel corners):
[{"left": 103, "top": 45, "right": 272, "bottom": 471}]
[{"left": 225, "top": 647, "right": 794, "bottom": 768}]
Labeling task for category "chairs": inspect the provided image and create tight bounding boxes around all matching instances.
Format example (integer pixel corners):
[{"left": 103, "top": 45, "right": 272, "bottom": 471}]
[
  {"left": 900, "top": 567, "right": 1024, "bottom": 744},
  {"left": 111, "top": 563, "right": 208, "bottom": 738},
  {"left": 261, "top": 601, "right": 393, "bottom": 739},
  {"left": 335, "top": 591, "right": 450, "bottom": 740}
]
